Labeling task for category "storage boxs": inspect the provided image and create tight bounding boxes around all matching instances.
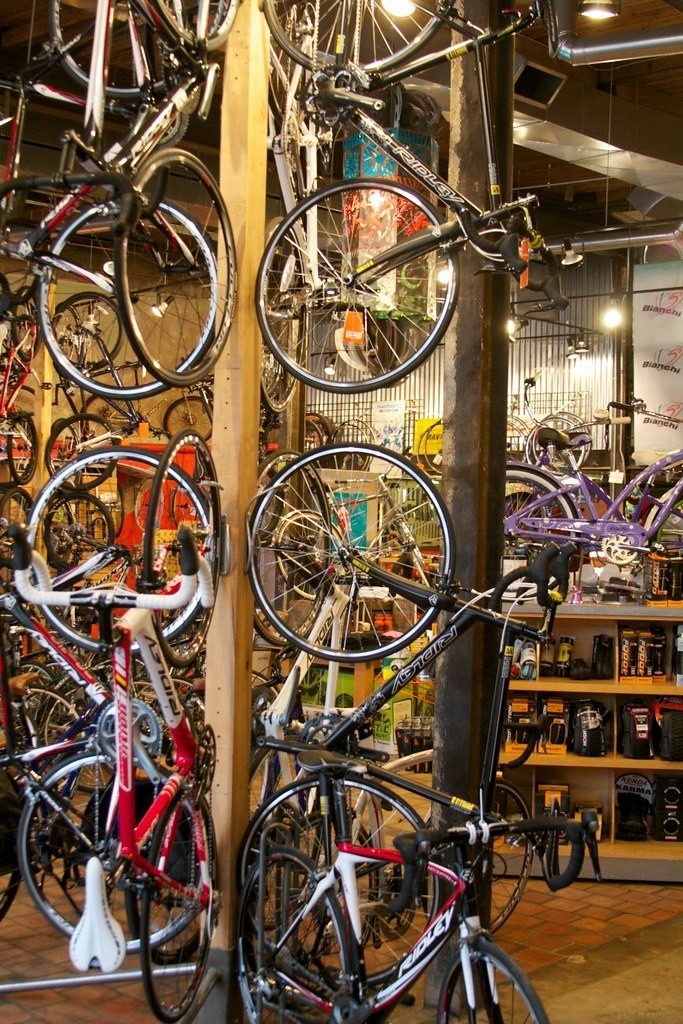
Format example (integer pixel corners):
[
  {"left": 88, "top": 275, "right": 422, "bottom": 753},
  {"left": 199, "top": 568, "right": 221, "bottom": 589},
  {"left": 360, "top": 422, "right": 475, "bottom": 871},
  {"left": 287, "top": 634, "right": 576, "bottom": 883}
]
[{"left": 304, "top": 669, "right": 435, "bottom": 755}]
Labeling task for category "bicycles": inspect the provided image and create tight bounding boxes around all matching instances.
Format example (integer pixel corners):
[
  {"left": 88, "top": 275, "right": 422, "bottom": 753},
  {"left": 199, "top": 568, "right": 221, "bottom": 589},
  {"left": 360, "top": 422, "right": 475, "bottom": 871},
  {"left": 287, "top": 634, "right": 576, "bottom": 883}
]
[
  {"left": 261, "top": 0, "right": 571, "bottom": 391},
  {"left": 0, "top": 0, "right": 683, "bottom": 1024}
]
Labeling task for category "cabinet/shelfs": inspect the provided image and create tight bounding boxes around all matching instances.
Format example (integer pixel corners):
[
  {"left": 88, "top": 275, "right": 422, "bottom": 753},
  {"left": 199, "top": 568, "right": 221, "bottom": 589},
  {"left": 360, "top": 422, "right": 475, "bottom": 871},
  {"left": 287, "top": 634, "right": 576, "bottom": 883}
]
[{"left": 350, "top": 603, "right": 683, "bottom": 883}]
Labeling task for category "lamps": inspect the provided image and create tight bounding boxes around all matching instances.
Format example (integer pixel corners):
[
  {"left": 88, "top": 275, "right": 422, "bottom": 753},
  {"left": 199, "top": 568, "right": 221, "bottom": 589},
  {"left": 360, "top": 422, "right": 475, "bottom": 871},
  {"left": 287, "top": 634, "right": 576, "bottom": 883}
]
[
  {"left": 324, "top": 356, "right": 336, "bottom": 375},
  {"left": 561, "top": 236, "right": 584, "bottom": 266},
  {"left": 565, "top": 337, "right": 580, "bottom": 359},
  {"left": 152, "top": 296, "right": 174, "bottom": 318},
  {"left": 507, "top": 320, "right": 531, "bottom": 333},
  {"left": 576, "top": 328, "right": 589, "bottom": 353},
  {"left": 602, "top": 289, "right": 627, "bottom": 327},
  {"left": 579, "top": 0, "right": 622, "bottom": 21}
]
